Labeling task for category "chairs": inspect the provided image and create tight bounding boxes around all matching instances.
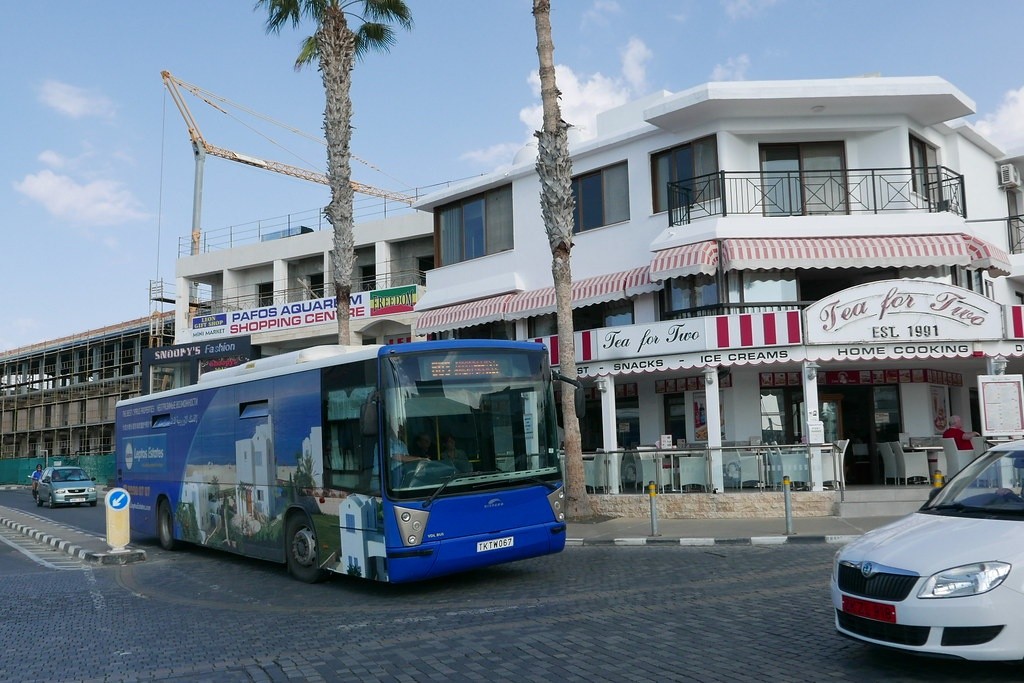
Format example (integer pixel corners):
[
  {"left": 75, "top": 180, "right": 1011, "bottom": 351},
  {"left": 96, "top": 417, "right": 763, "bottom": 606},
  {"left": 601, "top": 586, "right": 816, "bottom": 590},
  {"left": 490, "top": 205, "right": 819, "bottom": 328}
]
[
  {"left": 876, "top": 442, "right": 921, "bottom": 485},
  {"left": 939, "top": 437, "right": 980, "bottom": 487},
  {"left": 504, "top": 438, "right": 851, "bottom": 493},
  {"left": 889, "top": 441, "right": 931, "bottom": 486},
  {"left": 971, "top": 437, "right": 1018, "bottom": 489}
]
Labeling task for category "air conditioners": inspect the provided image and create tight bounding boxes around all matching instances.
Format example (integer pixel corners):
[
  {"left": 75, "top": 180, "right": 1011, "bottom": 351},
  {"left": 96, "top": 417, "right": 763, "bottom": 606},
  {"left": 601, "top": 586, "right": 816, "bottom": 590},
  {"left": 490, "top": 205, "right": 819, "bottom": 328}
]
[{"left": 1000, "top": 164, "right": 1020, "bottom": 188}]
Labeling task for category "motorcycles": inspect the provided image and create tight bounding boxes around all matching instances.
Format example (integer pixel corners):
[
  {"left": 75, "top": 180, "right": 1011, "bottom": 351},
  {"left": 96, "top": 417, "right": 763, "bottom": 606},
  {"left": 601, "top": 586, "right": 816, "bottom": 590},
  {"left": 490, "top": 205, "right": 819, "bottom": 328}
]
[{"left": 27, "top": 475, "right": 39, "bottom": 500}]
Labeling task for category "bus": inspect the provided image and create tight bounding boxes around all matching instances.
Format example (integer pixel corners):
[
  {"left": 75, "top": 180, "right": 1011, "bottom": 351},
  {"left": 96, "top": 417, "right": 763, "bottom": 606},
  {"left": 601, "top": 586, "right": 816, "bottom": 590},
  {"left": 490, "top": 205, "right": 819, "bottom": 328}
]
[{"left": 115, "top": 339, "right": 588, "bottom": 588}]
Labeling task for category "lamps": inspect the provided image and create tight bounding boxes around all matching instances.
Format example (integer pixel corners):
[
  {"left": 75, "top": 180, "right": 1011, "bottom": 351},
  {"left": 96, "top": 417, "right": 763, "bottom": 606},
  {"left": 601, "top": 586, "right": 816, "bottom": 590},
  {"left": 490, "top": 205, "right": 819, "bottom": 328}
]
[
  {"left": 806, "top": 362, "right": 821, "bottom": 380},
  {"left": 993, "top": 355, "right": 1010, "bottom": 375},
  {"left": 700, "top": 367, "right": 714, "bottom": 384},
  {"left": 594, "top": 376, "right": 607, "bottom": 393},
  {"left": 811, "top": 106, "right": 825, "bottom": 112}
]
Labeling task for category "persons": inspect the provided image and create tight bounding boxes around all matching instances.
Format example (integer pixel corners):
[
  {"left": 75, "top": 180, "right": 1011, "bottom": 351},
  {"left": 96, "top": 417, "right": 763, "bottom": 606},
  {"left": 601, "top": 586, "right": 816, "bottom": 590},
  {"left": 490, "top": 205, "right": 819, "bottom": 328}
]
[
  {"left": 370, "top": 419, "right": 431, "bottom": 490},
  {"left": 54, "top": 472, "right": 60, "bottom": 480},
  {"left": 943, "top": 415, "right": 980, "bottom": 450},
  {"left": 67, "top": 472, "right": 80, "bottom": 480},
  {"left": 411, "top": 433, "right": 437, "bottom": 459},
  {"left": 30, "top": 464, "right": 43, "bottom": 491},
  {"left": 996, "top": 484, "right": 1024, "bottom": 499},
  {"left": 440, "top": 435, "right": 466, "bottom": 461}
]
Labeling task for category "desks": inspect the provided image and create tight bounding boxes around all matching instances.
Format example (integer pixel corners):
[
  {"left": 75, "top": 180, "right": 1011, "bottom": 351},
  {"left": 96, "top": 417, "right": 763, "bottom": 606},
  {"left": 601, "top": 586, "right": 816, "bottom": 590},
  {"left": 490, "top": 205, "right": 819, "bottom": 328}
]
[
  {"left": 751, "top": 450, "right": 786, "bottom": 490},
  {"left": 655, "top": 451, "right": 704, "bottom": 492},
  {"left": 903, "top": 446, "right": 943, "bottom": 484},
  {"left": 560, "top": 454, "right": 595, "bottom": 458},
  {"left": 782, "top": 447, "right": 834, "bottom": 490}
]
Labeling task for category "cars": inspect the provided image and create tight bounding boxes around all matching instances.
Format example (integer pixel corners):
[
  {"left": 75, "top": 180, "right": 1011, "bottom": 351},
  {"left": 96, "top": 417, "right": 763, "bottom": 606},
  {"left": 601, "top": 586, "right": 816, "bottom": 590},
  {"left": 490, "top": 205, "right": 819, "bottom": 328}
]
[
  {"left": 37, "top": 467, "right": 98, "bottom": 510},
  {"left": 828, "top": 436, "right": 1024, "bottom": 662}
]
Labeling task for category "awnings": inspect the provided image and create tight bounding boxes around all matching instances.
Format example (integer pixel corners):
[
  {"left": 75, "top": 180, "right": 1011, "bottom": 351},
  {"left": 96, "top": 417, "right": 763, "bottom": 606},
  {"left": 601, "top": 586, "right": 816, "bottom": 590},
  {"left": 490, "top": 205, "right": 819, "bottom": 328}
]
[
  {"left": 963, "top": 235, "right": 1012, "bottom": 278},
  {"left": 415, "top": 266, "right": 664, "bottom": 337},
  {"left": 722, "top": 235, "right": 972, "bottom": 274},
  {"left": 649, "top": 241, "right": 718, "bottom": 282}
]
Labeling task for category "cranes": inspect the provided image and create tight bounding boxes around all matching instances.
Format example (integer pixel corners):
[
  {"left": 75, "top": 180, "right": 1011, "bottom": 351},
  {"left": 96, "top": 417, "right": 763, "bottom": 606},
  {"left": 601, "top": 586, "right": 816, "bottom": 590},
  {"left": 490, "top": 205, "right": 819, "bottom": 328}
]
[{"left": 160, "top": 69, "right": 421, "bottom": 332}]
